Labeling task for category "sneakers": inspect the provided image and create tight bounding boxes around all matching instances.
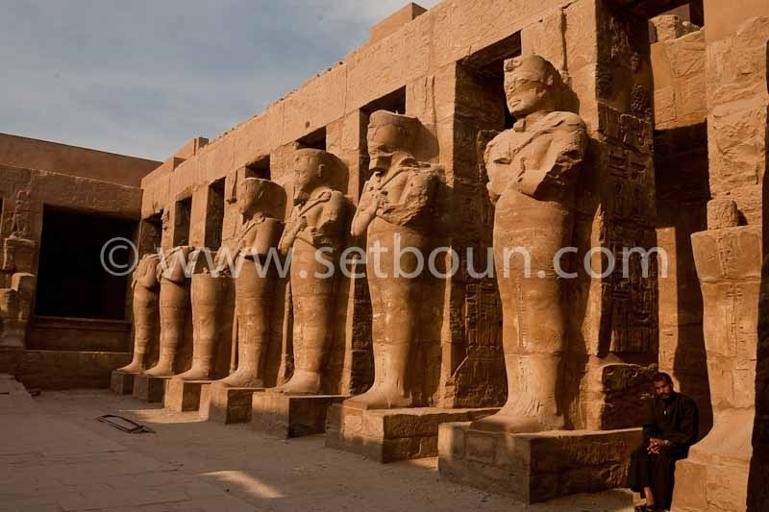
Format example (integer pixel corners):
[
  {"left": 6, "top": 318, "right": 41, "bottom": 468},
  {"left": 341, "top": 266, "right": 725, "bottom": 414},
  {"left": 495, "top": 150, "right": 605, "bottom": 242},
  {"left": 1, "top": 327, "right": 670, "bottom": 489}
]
[{"left": 634, "top": 502, "right": 657, "bottom": 512}]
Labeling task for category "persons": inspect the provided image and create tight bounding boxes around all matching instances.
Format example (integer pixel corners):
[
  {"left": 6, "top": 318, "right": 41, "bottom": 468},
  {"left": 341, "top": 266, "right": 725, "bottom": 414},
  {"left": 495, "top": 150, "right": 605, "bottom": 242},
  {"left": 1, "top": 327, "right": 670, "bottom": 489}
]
[
  {"left": 469, "top": 52, "right": 587, "bottom": 433},
  {"left": 143, "top": 245, "right": 197, "bottom": 376},
  {"left": 213, "top": 177, "right": 282, "bottom": 387},
  {"left": 625, "top": 371, "right": 700, "bottom": 512},
  {"left": 174, "top": 250, "right": 227, "bottom": 381},
  {"left": 341, "top": 108, "right": 446, "bottom": 410},
  {"left": 116, "top": 251, "right": 158, "bottom": 373},
  {"left": 265, "top": 146, "right": 349, "bottom": 395}
]
